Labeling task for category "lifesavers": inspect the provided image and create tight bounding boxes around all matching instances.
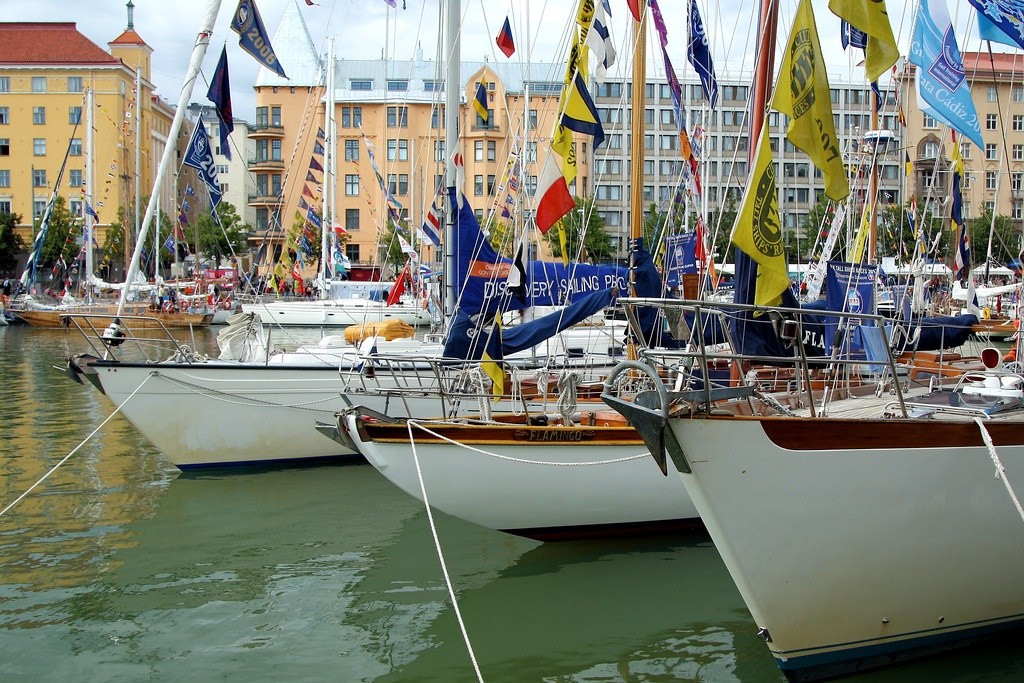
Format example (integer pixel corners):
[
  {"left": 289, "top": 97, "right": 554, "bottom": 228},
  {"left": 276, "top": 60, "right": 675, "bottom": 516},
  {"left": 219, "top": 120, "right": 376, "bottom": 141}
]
[
  {"left": 983, "top": 307, "right": 991, "bottom": 320},
  {"left": 163, "top": 301, "right": 174, "bottom": 311},
  {"left": 180, "top": 301, "right": 189, "bottom": 310},
  {"left": 223, "top": 300, "right": 231, "bottom": 310}
]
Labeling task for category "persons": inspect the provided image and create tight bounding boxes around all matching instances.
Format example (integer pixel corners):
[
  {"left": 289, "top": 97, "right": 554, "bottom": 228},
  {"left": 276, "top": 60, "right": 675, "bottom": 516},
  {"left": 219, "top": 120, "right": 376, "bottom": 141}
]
[
  {"left": 3, "top": 277, "right": 12, "bottom": 297},
  {"left": 158, "top": 283, "right": 166, "bottom": 310},
  {"left": 167, "top": 287, "right": 175, "bottom": 305}
]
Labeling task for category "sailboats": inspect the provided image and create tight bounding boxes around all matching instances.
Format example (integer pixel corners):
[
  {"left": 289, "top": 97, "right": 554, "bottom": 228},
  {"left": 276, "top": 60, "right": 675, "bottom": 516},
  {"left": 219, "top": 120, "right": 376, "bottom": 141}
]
[{"left": 7, "top": 3, "right": 1024, "bottom": 671}]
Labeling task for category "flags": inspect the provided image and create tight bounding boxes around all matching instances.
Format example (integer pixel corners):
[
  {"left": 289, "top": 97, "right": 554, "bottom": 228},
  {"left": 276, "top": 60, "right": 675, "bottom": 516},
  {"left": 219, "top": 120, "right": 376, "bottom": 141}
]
[{"left": 0, "top": 0, "right": 1024, "bottom": 402}]
